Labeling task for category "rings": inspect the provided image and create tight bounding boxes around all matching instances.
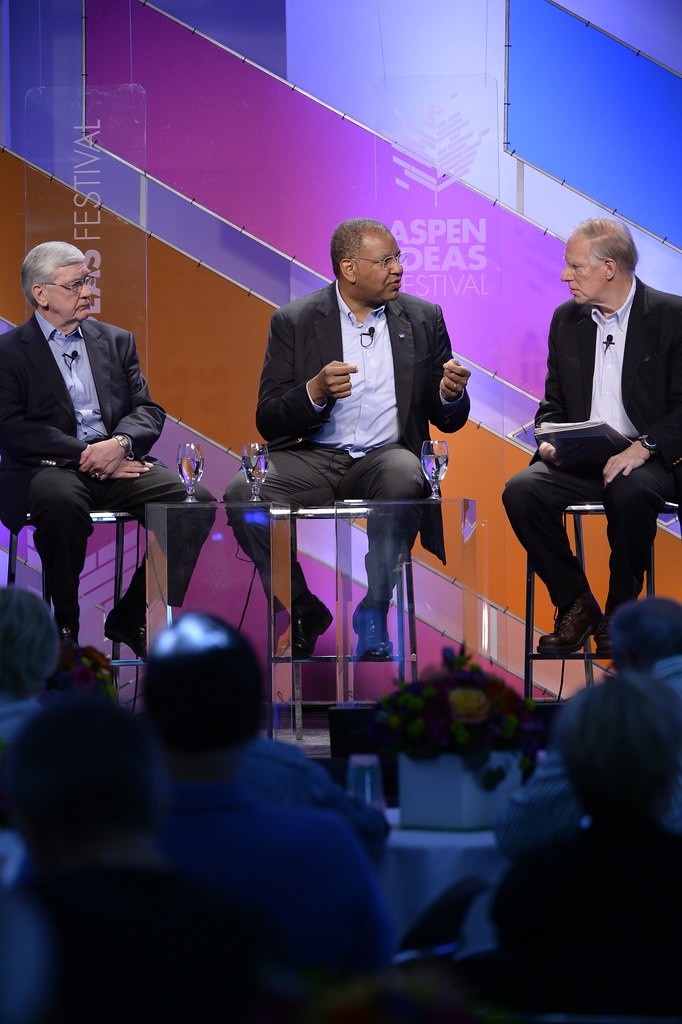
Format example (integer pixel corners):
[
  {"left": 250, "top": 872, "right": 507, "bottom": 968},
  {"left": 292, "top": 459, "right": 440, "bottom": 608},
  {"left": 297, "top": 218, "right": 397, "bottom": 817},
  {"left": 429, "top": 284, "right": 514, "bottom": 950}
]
[
  {"left": 95, "top": 473, "right": 98, "bottom": 479},
  {"left": 451, "top": 384, "right": 456, "bottom": 391}
]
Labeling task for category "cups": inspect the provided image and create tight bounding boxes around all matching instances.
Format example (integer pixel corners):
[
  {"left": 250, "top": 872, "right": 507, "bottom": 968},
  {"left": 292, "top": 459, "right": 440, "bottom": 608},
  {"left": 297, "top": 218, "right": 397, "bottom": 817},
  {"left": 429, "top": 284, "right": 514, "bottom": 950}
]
[{"left": 346, "top": 755, "right": 386, "bottom": 813}]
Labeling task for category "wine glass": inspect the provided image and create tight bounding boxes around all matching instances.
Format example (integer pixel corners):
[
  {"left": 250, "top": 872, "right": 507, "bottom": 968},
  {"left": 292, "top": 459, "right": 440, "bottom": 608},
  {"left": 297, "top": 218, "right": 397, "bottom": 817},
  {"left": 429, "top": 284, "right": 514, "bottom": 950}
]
[
  {"left": 241, "top": 441, "right": 269, "bottom": 501},
  {"left": 176, "top": 442, "right": 204, "bottom": 503},
  {"left": 421, "top": 440, "right": 449, "bottom": 499}
]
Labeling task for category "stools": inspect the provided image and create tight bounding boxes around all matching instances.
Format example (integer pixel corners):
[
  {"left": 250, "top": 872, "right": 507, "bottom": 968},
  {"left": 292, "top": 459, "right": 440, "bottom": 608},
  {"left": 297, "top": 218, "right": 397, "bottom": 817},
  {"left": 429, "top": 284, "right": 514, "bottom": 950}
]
[
  {"left": 524, "top": 503, "right": 679, "bottom": 702},
  {"left": 265, "top": 501, "right": 432, "bottom": 739},
  {"left": 5, "top": 511, "right": 172, "bottom": 665}
]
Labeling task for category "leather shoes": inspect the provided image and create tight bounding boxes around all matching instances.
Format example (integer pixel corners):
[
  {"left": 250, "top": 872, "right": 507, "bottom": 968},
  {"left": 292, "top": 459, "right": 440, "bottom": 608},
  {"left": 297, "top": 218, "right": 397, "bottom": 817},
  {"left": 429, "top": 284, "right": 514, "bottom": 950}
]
[
  {"left": 352, "top": 600, "right": 394, "bottom": 661},
  {"left": 292, "top": 594, "right": 334, "bottom": 660},
  {"left": 52, "top": 603, "right": 82, "bottom": 648},
  {"left": 595, "top": 619, "right": 618, "bottom": 661},
  {"left": 536, "top": 597, "right": 601, "bottom": 656},
  {"left": 102, "top": 605, "right": 147, "bottom": 660}
]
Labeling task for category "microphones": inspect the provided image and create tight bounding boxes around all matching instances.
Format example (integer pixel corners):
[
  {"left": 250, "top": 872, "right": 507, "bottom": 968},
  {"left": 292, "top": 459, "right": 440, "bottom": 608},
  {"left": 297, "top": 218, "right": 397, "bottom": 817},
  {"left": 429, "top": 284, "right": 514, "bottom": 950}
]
[
  {"left": 369, "top": 326, "right": 375, "bottom": 338},
  {"left": 72, "top": 350, "right": 78, "bottom": 360},
  {"left": 606, "top": 335, "right": 613, "bottom": 347}
]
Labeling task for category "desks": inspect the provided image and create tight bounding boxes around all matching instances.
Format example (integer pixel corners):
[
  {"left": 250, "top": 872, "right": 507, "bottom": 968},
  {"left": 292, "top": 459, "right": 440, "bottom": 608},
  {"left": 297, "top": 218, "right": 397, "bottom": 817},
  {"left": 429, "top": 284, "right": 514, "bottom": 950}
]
[{"left": 145, "top": 498, "right": 478, "bottom": 740}]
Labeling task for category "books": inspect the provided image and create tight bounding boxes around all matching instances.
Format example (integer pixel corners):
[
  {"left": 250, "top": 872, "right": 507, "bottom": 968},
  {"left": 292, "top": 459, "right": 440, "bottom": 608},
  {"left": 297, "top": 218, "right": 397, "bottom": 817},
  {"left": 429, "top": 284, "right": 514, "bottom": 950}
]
[{"left": 532, "top": 421, "right": 634, "bottom": 472}]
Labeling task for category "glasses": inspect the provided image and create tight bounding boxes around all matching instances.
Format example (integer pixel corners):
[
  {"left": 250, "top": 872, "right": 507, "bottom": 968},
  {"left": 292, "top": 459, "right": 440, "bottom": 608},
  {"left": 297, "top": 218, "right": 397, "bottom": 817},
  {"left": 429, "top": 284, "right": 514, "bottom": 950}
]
[
  {"left": 41, "top": 271, "right": 98, "bottom": 297},
  {"left": 352, "top": 248, "right": 409, "bottom": 270}
]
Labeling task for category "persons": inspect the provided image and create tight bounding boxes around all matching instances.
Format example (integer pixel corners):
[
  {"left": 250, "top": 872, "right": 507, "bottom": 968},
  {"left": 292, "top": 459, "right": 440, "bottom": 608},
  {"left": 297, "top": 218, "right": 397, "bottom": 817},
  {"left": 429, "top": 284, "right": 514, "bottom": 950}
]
[
  {"left": 502, "top": 218, "right": 682, "bottom": 654},
  {"left": 0, "top": 242, "right": 219, "bottom": 659},
  {"left": 223, "top": 217, "right": 471, "bottom": 659},
  {"left": 0, "top": 587, "right": 401, "bottom": 1024},
  {"left": 493, "top": 598, "right": 682, "bottom": 1024}
]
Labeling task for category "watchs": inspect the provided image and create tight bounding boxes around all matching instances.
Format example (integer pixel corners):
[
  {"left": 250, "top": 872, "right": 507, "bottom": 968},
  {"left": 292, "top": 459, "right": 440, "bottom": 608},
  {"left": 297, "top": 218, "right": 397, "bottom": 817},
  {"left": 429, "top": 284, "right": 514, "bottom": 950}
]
[
  {"left": 639, "top": 434, "right": 658, "bottom": 454},
  {"left": 116, "top": 435, "right": 129, "bottom": 453}
]
